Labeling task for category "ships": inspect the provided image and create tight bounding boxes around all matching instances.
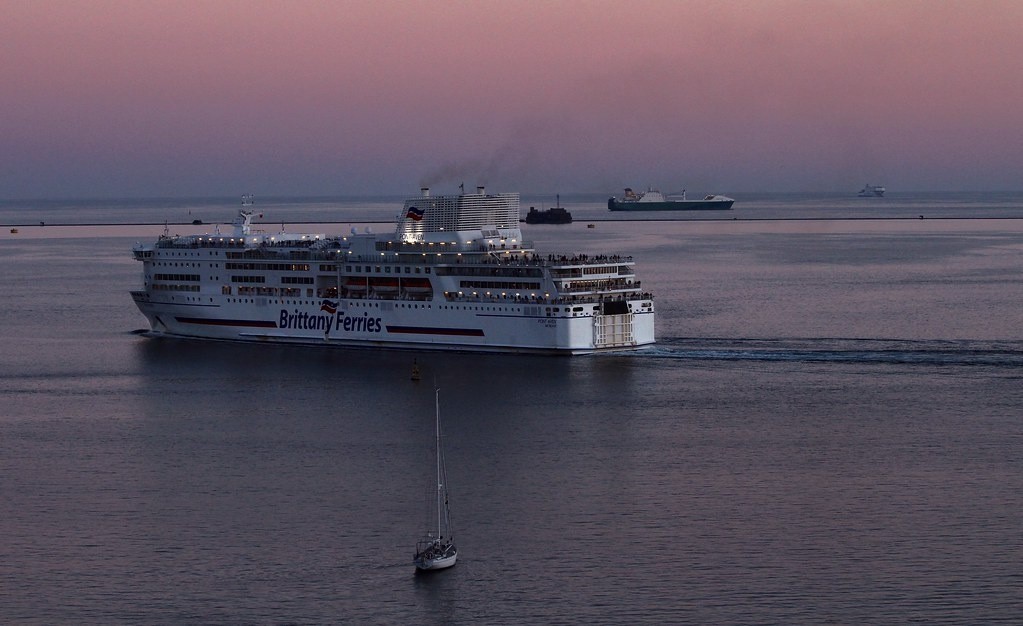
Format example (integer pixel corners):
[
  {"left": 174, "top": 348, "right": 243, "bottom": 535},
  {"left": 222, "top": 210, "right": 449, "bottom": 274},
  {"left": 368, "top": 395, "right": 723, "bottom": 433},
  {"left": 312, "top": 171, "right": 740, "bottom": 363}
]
[{"left": 128, "top": 186, "right": 658, "bottom": 358}]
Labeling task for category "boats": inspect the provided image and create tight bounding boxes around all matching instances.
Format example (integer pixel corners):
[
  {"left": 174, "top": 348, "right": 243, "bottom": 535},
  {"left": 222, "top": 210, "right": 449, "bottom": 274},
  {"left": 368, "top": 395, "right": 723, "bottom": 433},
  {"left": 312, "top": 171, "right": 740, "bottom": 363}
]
[
  {"left": 858, "top": 183, "right": 886, "bottom": 199},
  {"left": 343, "top": 280, "right": 367, "bottom": 292},
  {"left": 525, "top": 194, "right": 573, "bottom": 225},
  {"left": 402, "top": 281, "right": 432, "bottom": 295},
  {"left": 606, "top": 183, "right": 736, "bottom": 212},
  {"left": 371, "top": 280, "right": 399, "bottom": 293}
]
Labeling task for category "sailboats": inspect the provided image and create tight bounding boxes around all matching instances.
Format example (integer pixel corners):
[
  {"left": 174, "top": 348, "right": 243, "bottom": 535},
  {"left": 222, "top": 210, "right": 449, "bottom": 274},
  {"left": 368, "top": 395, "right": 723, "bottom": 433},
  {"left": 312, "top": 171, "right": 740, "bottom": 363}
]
[{"left": 412, "top": 388, "right": 460, "bottom": 573}]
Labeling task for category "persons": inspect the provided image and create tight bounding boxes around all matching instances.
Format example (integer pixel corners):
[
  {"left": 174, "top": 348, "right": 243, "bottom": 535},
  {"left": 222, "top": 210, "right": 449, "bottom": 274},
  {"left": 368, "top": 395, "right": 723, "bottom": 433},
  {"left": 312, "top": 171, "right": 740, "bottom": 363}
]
[
  {"left": 625, "top": 280, "right": 641, "bottom": 288},
  {"left": 515, "top": 295, "right": 622, "bottom": 303},
  {"left": 487, "top": 253, "right": 631, "bottom": 265},
  {"left": 191, "top": 240, "right": 244, "bottom": 248},
  {"left": 630, "top": 291, "right": 652, "bottom": 299},
  {"left": 272, "top": 238, "right": 340, "bottom": 248}
]
